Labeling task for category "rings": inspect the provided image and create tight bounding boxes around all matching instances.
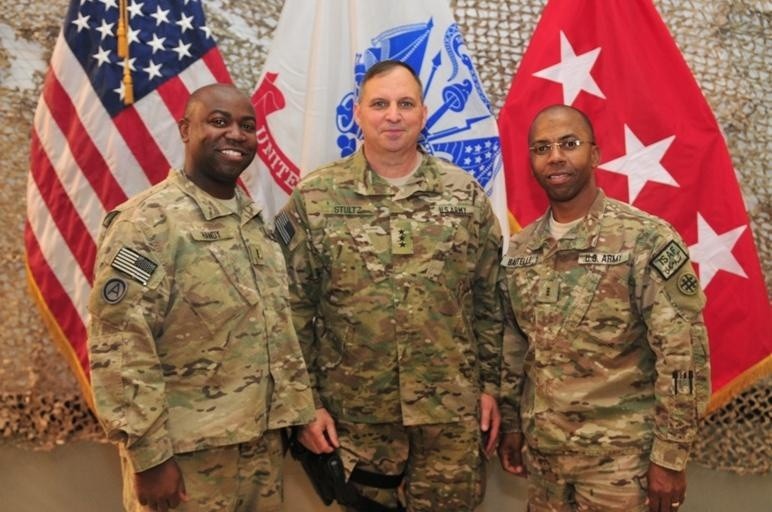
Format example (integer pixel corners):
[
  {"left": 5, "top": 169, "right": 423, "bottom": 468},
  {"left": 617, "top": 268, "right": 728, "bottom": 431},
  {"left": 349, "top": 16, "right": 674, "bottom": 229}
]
[{"left": 671, "top": 500, "right": 681, "bottom": 507}]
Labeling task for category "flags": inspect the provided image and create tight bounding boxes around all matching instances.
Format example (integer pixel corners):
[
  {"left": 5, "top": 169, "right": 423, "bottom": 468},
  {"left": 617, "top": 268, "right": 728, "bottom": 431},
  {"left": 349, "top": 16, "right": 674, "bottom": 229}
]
[
  {"left": 238, "top": 2, "right": 511, "bottom": 255},
  {"left": 493, "top": 2, "right": 772, "bottom": 424},
  {"left": 23, "top": 1, "right": 255, "bottom": 448}
]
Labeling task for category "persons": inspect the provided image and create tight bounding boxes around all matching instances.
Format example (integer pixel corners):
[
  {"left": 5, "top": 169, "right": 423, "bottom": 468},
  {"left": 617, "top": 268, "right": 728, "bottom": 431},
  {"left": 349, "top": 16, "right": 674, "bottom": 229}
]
[
  {"left": 273, "top": 62, "right": 506, "bottom": 512},
  {"left": 83, "top": 83, "right": 317, "bottom": 512},
  {"left": 495, "top": 103, "right": 712, "bottom": 512}
]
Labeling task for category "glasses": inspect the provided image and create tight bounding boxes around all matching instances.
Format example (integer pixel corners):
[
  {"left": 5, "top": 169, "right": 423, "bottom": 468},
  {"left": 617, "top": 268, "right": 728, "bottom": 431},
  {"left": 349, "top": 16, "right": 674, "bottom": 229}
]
[{"left": 528, "top": 139, "right": 596, "bottom": 154}]
